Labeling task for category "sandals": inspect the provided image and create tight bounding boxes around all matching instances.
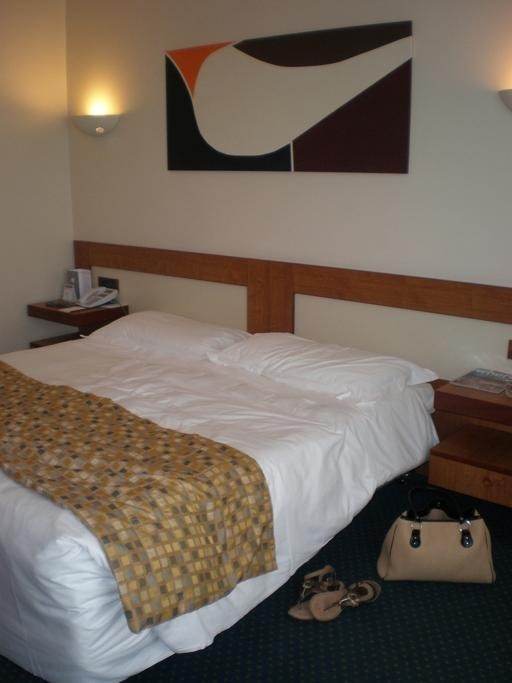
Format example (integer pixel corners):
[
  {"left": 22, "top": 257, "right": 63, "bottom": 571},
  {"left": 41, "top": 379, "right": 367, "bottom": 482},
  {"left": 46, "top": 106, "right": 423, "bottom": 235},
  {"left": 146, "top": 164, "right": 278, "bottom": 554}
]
[
  {"left": 311, "top": 577, "right": 381, "bottom": 625},
  {"left": 287, "top": 566, "right": 344, "bottom": 624}
]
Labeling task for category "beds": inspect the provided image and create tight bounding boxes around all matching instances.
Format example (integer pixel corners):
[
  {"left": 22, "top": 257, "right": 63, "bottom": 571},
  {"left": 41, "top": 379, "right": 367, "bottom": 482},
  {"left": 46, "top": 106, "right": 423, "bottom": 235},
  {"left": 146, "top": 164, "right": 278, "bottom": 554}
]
[{"left": 0, "top": 239, "right": 512, "bottom": 683}]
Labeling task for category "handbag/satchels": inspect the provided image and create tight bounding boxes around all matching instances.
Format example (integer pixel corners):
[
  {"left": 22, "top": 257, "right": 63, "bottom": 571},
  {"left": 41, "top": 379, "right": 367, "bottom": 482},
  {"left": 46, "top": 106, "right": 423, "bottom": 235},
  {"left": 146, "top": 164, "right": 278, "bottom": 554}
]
[{"left": 377, "top": 486, "right": 497, "bottom": 586}]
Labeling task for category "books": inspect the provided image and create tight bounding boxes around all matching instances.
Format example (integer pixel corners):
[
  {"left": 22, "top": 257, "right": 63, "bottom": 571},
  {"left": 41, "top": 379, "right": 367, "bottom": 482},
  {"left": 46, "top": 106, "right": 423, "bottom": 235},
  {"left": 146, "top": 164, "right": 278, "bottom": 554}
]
[{"left": 447, "top": 367, "right": 512, "bottom": 394}]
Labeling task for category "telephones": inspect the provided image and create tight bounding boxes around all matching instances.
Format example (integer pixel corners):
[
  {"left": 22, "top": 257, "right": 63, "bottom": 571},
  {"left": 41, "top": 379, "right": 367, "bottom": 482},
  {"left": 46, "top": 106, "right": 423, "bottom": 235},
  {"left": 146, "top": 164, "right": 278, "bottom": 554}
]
[{"left": 75, "top": 287, "right": 118, "bottom": 308}]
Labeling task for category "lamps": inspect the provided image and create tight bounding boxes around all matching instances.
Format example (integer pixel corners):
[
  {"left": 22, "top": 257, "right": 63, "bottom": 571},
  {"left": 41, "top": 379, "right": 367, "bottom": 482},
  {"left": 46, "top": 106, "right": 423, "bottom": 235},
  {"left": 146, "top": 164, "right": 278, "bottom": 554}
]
[
  {"left": 497, "top": 89, "right": 512, "bottom": 115},
  {"left": 71, "top": 92, "right": 121, "bottom": 137}
]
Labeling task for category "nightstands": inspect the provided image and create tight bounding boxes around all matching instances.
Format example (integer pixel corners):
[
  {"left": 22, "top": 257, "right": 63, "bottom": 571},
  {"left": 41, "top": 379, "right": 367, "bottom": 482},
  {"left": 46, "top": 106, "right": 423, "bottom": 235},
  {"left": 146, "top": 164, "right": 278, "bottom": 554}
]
[
  {"left": 27, "top": 300, "right": 129, "bottom": 349},
  {"left": 426, "top": 368, "right": 511, "bottom": 508}
]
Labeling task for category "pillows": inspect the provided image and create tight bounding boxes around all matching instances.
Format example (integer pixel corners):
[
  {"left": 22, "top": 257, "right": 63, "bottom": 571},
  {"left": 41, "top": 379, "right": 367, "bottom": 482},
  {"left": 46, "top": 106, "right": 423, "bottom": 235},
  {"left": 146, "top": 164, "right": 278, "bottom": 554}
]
[
  {"left": 206, "top": 331, "right": 439, "bottom": 406},
  {"left": 80, "top": 310, "right": 252, "bottom": 361}
]
